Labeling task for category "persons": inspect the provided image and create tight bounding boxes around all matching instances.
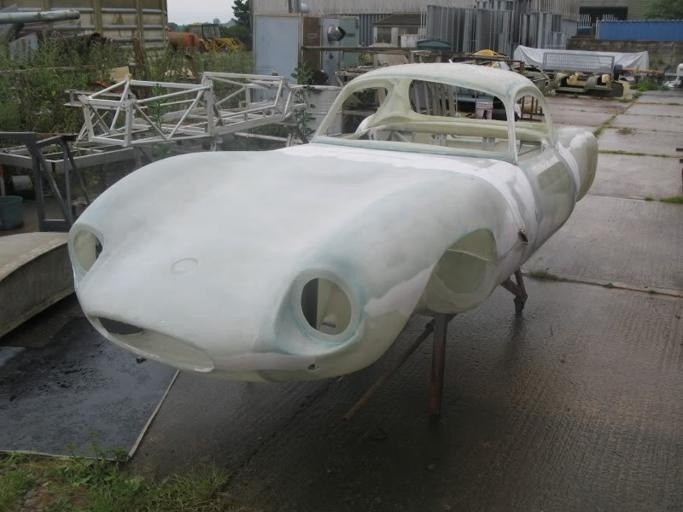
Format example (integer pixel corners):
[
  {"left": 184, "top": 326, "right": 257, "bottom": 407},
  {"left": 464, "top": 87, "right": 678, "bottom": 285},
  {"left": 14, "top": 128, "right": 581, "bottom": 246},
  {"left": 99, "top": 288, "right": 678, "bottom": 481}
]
[
  {"left": 492, "top": 52, "right": 510, "bottom": 70},
  {"left": 475, "top": 90, "right": 495, "bottom": 119},
  {"left": 676, "top": 61, "right": 683, "bottom": 88}
]
